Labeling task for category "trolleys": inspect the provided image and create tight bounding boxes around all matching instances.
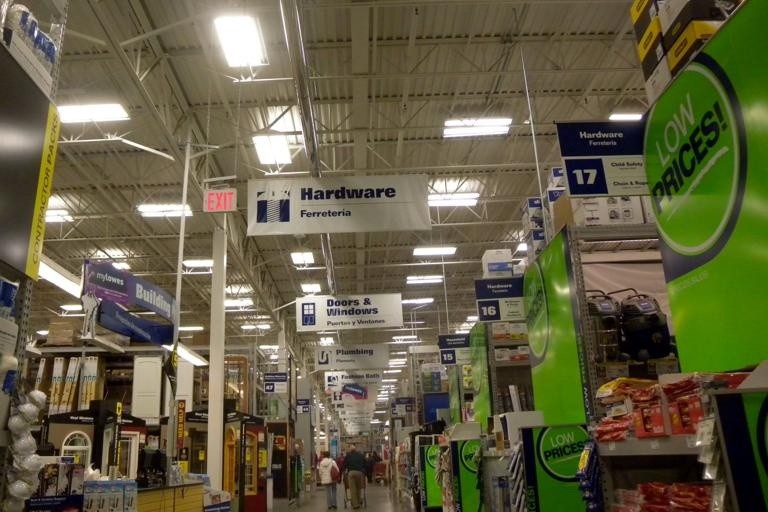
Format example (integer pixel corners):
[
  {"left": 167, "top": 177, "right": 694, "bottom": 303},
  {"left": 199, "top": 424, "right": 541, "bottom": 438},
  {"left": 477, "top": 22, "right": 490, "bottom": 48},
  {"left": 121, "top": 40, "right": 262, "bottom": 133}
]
[{"left": 343, "top": 471, "right": 367, "bottom": 509}]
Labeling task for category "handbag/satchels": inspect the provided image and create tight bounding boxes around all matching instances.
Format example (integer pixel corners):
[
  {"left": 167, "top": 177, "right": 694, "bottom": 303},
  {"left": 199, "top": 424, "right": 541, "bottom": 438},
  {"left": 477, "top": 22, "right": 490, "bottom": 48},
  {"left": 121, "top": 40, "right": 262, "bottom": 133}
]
[{"left": 331, "top": 467, "right": 339, "bottom": 481}]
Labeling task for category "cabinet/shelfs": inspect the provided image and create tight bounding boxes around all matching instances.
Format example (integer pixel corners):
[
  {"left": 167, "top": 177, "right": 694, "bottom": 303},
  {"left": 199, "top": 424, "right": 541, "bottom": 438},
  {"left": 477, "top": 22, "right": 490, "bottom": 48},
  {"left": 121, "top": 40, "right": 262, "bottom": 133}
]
[{"left": 468, "top": 222, "right": 734, "bottom": 511}]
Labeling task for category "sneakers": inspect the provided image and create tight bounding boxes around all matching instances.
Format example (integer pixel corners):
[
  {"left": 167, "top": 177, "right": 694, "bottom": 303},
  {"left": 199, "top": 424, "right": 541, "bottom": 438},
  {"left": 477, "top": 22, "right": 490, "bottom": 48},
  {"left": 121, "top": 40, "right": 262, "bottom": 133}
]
[{"left": 328, "top": 505, "right": 337, "bottom": 509}]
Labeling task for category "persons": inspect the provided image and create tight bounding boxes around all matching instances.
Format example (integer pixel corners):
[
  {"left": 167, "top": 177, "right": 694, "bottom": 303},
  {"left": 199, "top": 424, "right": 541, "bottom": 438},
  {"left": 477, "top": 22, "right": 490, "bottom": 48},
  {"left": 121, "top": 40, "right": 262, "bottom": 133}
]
[
  {"left": 341, "top": 445, "right": 368, "bottom": 510},
  {"left": 317, "top": 451, "right": 340, "bottom": 510},
  {"left": 318, "top": 450, "right": 380, "bottom": 484}
]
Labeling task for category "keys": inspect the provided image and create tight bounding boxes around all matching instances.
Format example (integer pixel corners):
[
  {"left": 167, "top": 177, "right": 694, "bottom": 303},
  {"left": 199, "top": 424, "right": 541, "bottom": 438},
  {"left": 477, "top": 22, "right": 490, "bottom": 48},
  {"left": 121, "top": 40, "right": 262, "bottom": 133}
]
[
  {"left": 80, "top": 290, "right": 98, "bottom": 337},
  {"left": 89, "top": 293, "right": 101, "bottom": 340}
]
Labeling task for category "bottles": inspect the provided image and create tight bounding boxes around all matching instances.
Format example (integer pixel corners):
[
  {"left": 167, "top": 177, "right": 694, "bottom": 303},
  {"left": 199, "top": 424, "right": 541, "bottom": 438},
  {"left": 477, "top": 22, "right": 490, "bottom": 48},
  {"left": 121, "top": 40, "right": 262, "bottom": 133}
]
[{"left": 108, "top": 462, "right": 117, "bottom": 480}]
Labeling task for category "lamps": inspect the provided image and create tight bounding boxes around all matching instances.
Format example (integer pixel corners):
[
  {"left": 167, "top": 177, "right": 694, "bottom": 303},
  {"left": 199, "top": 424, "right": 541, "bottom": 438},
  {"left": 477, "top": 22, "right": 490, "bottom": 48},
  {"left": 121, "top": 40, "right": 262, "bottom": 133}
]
[
  {"left": 161, "top": 341, "right": 210, "bottom": 367},
  {"left": 38, "top": 254, "right": 83, "bottom": 300}
]
